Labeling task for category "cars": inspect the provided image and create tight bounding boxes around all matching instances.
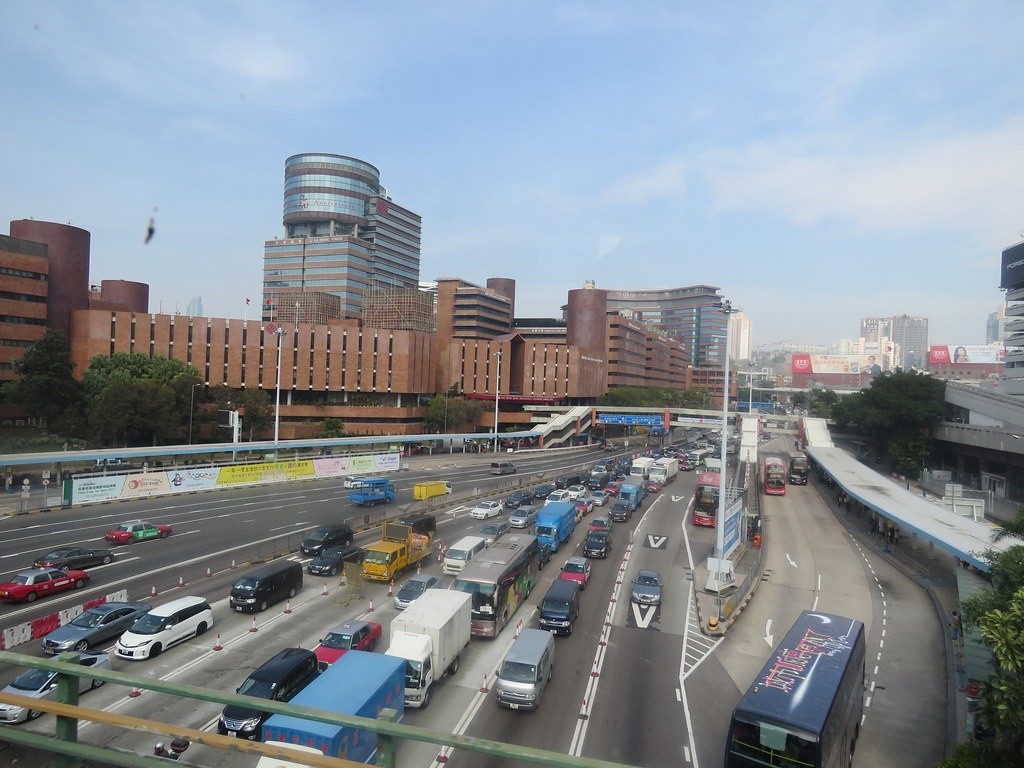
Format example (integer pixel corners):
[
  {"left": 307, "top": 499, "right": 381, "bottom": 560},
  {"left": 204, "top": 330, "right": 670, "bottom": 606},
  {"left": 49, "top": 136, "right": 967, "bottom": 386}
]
[
  {"left": 308, "top": 545, "right": 364, "bottom": 577},
  {"left": 41, "top": 601, "right": 152, "bottom": 655},
  {"left": 0, "top": 566, "right": 91, "bottom": 604},
  {"left": 0, "top": 649, "right": 114, "bottom": 725},
  {"left": 631, "top": 569, "right": 664, "bottom": 605},
  {"left": 559, "top": 556, "right": 592, "bottom": 591},
  {"left": 299, "top": 524, "right": 354, "bottom": 558},
  {"left": 313, "top": 619, "right": 382, "bottom": 673},
  {"left": 32, "top": 546, "right": 115, "bottom": 572},
  {"left": 394, "top": 573, "right": 442, "bottom": 610},
  {"left": 468, "top": 427, "right": 772, "bottom": 571},
  {"left": 105, "top": 519, "right": 173, "bottom": 545}
]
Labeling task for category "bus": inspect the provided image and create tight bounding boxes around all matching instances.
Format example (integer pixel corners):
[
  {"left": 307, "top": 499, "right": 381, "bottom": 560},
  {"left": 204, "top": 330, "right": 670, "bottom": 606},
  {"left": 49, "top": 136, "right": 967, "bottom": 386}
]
[
  {"left": 692, "top": 471, "right": 720, "bottom": 527},
  {"left": 788, "top": 451, "right": 808, "bottom": 485},
  {"left": 449, "top": 533, "right": 539, "bottom": 639},
  {"left": 764, "top": 457, "right": 785, "bottom": 496},
  {"left": 723, "top": 610, "right": 866, "bottom": 768}
]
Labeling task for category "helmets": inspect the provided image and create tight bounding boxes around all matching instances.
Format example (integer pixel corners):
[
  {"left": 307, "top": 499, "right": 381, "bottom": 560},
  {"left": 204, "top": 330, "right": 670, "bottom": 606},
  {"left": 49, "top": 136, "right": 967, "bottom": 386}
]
[{"left": 155, "top": 742, "right": 164, "bottom": 752}]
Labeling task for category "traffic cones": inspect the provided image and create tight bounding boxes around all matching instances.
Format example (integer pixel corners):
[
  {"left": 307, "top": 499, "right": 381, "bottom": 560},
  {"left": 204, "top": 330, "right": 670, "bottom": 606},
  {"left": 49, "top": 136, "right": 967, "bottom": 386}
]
[
  {"left": 177, "top": 574, "right": 185, "bottom": 588},
  {"left": 322, "top": 583, "right": 330, "bottom": 596},
  {"left": 248, "top": 615, "right": 259, "bottom": 633},
  {"left": 206, "top": 566, "right": 213, "bottom": 577},
  {"left": 437, "top": 543, "right": 448, "bottom": 564},
  {"left": 416, "top": 562, "right": 422, "bottom": 575},
  {"left": 284, "top": 599, "right": 292, "bottom": 613},
  {"left": 338, "top": 574, "right": 346, "bottom": 586},
  {"left": 128, "top": 686, "right": 141, "bottom": 698},
  {"left": 479, "top": 674, "right": 490, "bottom": 694},
  {"left": 149, "top": 584, "right": 158, "bottom": 598},
  {"left": 212, "top": 633, "right": 223, "bottom": 651},
  {"left": 367, "top": 598, "right": 375, "bottom": 612},
  {"left": 513, "top": 619, "right": 524, "bottom": 640},
  {"left": 231, "top": 559, "right": 237, "bottom": 569},
  {"left": 387, "top": 585, "right": 394, "bottom": 597},
  {"left": 436, "top": 743, "right": 449, "bottom": 763}
]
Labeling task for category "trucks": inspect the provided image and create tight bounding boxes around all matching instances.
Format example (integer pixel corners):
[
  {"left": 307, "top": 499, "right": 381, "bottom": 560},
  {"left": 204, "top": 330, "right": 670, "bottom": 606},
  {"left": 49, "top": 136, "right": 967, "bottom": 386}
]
[
  {"left": 412, "top": 480, "right": 453, "bottom": 500},
  {"left": 255, "top": 649, "right": 407, "bottom": 768},
  {"left": 349, "top": 478, "right": 399, "bottom": 508},
  {"left": 618, "top": 477, "right": 646, "bottom": 512},
  {"left": 528, "top": 502, "right": 575, "bottom": 553},
  {"left": 630, "top": 457, "right": 656, "bottom": 481},
  {"left": 362, "top": 521, "right": 434, "bottom": 583},
  {"left": 383, "top": 588, "right": 472, "bottom": 710},
  {"left": 647, "top": 457, "right": 678, "bottom": 487}
]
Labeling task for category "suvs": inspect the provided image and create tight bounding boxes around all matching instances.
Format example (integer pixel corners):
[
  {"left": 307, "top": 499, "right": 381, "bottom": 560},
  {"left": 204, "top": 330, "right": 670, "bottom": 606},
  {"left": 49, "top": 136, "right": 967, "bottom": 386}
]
[
  {"left": 217, "top": 647, "right": 323, "bottom": 742},
  {"left": 113, "top": 596, "right": 214, "bottom": 661}
]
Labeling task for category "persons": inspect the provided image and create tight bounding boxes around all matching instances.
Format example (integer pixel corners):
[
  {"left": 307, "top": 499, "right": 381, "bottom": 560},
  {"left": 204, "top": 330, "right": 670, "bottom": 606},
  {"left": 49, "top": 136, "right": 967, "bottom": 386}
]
[
  {"left": 172, "top": 473, "right": 183, "bottom": 486},
  {"left": 888, "top": 527, "right": 900, "bottom": 545},
  {"left": 859, "top": 355, "right": 882, "bottom": 376},
  {"left": 953, "top": 346, "right": 970, "bottom": 364},
  {"left": 951, "top": 611, "right": 960, "bottom": 640},
  {"left": 838, "top": 494, "right": 848, "bottom": 507},
  {"left": 154, "top": 742, "right": 169, "bottom": 759}
]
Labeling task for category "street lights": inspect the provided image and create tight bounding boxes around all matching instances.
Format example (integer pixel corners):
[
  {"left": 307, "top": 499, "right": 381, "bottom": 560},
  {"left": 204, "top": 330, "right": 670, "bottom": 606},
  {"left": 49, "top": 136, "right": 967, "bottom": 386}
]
[
  {"left": 748, "top": 361, "right": 757, "bottom": 416},
  {"left": 493, "top": 351, "right": 502, "bottom": 452},
  {"left": 273, "top": 327, "right": 283, "bottom": 464},
  {"left": 189, "top": 383, "right": 201, "bottom": 445},
  {"left": 715, "top": 299, "right": 740, "bottom": 560}
]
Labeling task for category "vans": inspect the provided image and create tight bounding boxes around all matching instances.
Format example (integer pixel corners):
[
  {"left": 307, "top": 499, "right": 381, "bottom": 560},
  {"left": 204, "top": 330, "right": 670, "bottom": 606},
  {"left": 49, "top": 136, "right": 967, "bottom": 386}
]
[
  {"left": 491, "top": 462, "right": 516, "bottom": 475},
  {"left": 494, "top": 628, "right": 556, "bottom": 711},
  {"left": 230, "top": 559, "right": 304, "bottom": 614},
  {"left": 536, "top": 578, "right": 580, "bottom": 637}
]
